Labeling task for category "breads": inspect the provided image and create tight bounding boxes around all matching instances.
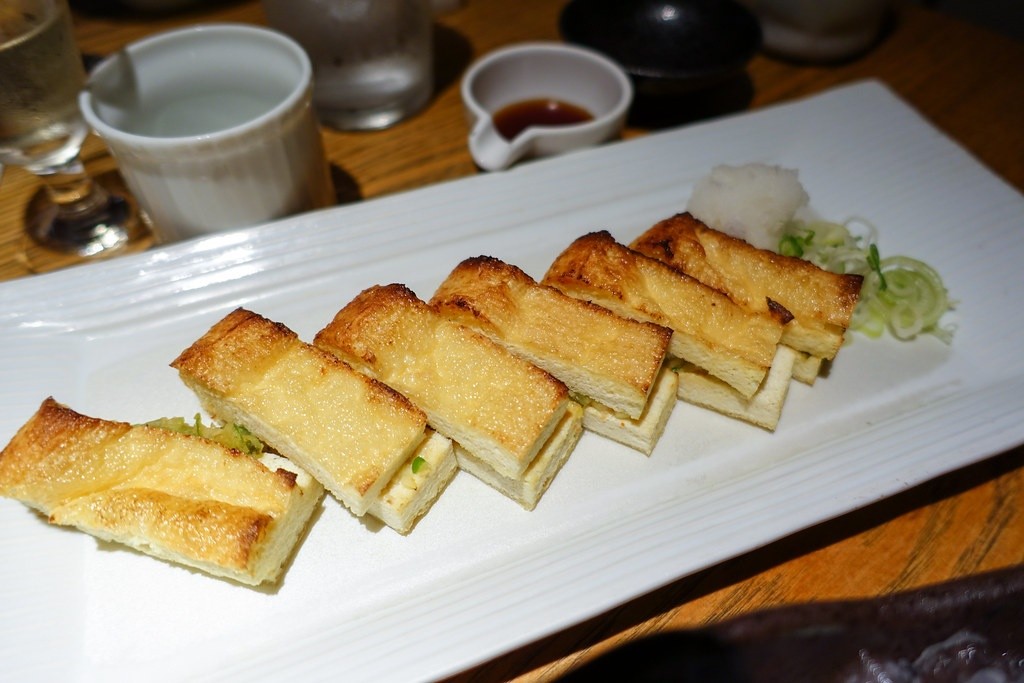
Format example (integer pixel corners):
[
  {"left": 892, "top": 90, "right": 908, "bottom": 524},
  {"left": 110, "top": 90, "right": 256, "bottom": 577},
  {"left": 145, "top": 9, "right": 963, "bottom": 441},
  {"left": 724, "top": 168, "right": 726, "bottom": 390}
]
[{"left": 0, "top": 213, "right": 867, "bottom": 585}]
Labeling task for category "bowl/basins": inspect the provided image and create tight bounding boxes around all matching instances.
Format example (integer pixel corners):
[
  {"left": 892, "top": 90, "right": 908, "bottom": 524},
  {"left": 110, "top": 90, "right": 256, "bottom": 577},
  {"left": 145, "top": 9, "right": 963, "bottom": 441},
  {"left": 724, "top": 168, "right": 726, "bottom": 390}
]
[
  {"left": 557, "top": 0, "right": 759, "bottom": 130},
  {"left": 459, "top": 43, "right": 632, "bottom": 170},
  {"left": 754, "top": 0, "right": 880, "bottom": 61}
]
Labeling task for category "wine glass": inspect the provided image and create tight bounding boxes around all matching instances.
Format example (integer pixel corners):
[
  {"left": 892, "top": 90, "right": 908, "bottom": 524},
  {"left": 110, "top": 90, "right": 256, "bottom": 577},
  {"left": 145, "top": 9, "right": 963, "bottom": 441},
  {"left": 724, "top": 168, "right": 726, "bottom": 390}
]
[{"left": 0, "top": 1, "right": 146, "bottom": 253}]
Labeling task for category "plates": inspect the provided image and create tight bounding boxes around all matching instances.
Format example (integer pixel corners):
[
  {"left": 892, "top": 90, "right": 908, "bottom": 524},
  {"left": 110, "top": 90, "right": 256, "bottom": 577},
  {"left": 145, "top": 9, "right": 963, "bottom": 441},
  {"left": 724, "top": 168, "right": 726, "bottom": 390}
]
[{"left": 0, "top": 79, "right": 1024, "bottom": 681}]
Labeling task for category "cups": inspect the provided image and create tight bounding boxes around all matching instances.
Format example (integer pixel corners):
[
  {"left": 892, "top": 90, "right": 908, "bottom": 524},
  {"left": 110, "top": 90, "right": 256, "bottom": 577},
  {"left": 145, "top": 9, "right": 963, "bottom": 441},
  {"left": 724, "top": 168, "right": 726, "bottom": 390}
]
[
  {"left": 262, "top": 0, "right": 434, "bottom": 131},
  {"left": 80, "top": 23, "right": 334, "bottom": 241}
]
